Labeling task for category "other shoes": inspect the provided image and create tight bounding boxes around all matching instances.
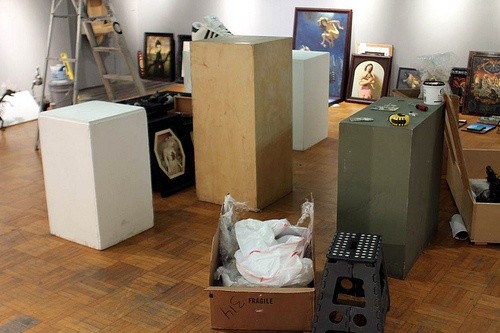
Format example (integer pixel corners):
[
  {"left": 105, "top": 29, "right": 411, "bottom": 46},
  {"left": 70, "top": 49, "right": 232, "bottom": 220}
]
[{"left": 192, "top": 15, "right": 233, "bottom": 40}]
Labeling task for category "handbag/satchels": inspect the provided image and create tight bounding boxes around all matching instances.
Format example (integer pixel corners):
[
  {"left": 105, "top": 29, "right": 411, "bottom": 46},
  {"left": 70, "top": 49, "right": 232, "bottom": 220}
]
[
  {"left": 234, "top": 218, "right": 314, "bottom": 287},
  {"left": 0, "top": 89, "right": 41, "bottom": 127}
]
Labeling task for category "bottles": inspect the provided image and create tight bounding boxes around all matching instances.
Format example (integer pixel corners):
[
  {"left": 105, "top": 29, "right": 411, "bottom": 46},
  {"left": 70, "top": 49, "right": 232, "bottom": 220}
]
[{"left": 486, "top": 166, "right": 500, "bottom": 203}]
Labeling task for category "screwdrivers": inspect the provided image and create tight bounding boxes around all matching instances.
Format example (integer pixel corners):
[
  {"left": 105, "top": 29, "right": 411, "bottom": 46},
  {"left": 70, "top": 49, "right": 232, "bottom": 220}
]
[{"left": 408, "top": 104, "right": 429, "bottom": 111}]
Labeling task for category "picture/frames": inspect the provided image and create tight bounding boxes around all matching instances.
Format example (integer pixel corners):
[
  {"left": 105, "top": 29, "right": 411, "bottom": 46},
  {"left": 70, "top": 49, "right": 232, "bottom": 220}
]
[
  {"left": 143, "top": 32, "right": 192, "bottom": 84},
  {"left": 292, "top": 7, "right": 500, "bottom": 118}
]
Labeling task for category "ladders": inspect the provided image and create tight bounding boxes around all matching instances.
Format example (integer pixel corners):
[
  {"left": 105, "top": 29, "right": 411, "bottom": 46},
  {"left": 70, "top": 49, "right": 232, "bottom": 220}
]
[{"left": 35, "top": 0, "right": 147, "bottom": 150}]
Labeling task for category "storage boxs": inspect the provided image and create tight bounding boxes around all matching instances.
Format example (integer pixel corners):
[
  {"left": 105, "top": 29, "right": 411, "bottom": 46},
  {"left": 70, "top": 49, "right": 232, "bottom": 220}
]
[
  {"left": 204, "top": 192, "right": 315, "bottom": 330},
  {"left": 445, "top": 149, "right": 500, "bottom": 246}
]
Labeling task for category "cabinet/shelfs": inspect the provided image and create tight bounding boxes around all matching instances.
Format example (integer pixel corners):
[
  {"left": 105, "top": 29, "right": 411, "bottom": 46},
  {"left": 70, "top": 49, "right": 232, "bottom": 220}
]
[
  {"left": 38, "top": 100, "right": 155, "bottom": 251},
  {"left": 336, "top": 97, "right": 446, "bottom": 281},
  {"left": 189, "top": 35, "right": 291, "bottom": 212},
  {"left": 292, "top": 50, "right": 331, "bottom": 150}
]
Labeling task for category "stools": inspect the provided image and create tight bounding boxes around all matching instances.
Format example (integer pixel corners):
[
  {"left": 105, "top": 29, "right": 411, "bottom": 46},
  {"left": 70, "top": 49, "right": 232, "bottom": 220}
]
[{"left": 311, "top": 231, "right": 391, "bottom": 333}]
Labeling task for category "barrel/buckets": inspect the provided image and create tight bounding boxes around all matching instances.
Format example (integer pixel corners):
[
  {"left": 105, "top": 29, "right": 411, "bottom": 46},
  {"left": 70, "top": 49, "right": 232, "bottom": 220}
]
[
  {"left": 49, "top": 80, "right": 73, "bottom": 109},
  {"left": 423, "top": 81, "right": 445, "bottom": 105}
]
